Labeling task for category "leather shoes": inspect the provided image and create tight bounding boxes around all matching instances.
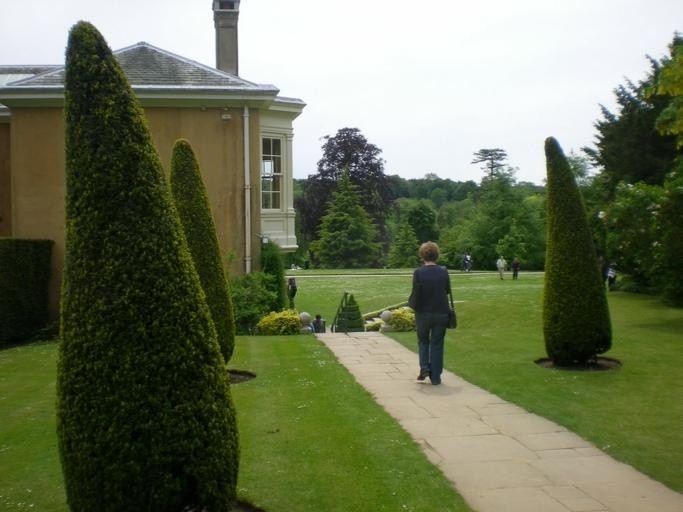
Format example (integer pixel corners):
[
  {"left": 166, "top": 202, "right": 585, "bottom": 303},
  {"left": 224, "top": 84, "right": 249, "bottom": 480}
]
[{"left": 417, "top": 369, "right": 442, "bottom": 384}]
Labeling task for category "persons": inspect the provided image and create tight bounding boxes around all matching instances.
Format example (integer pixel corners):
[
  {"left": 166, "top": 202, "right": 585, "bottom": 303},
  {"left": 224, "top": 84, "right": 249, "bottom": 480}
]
[
  {"left": 311, "top": 314, "right": 326, "bottom": 333},
  {"left": 286, "top": 279, "right": 297, "bottom": 309},
  {"left": 599, "top": 255, "right": 608, "bottom": 283},
  {"left": 607, "top": 256, "right": 617, "bottom": 291},
  {"left": 511, "top": 256, "right": 518, "bottom": 280},
  {"left": 495, "top": 254, "right": 507, "bottom": 280},
  {"left": 462, "top": 252, "right": 473, "bottom": 272},
  {"left": 406, "top": 240, "right": 451, "bottom": 386},
  {"left": 516, "top": 262, "right": 521, "bottom": 280}
]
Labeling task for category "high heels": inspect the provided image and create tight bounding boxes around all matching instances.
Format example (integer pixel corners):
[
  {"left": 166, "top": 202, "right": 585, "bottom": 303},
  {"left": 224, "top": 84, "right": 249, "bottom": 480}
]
[{"left": 449, "top": 310, "right": 457, "bottom": 329}]
